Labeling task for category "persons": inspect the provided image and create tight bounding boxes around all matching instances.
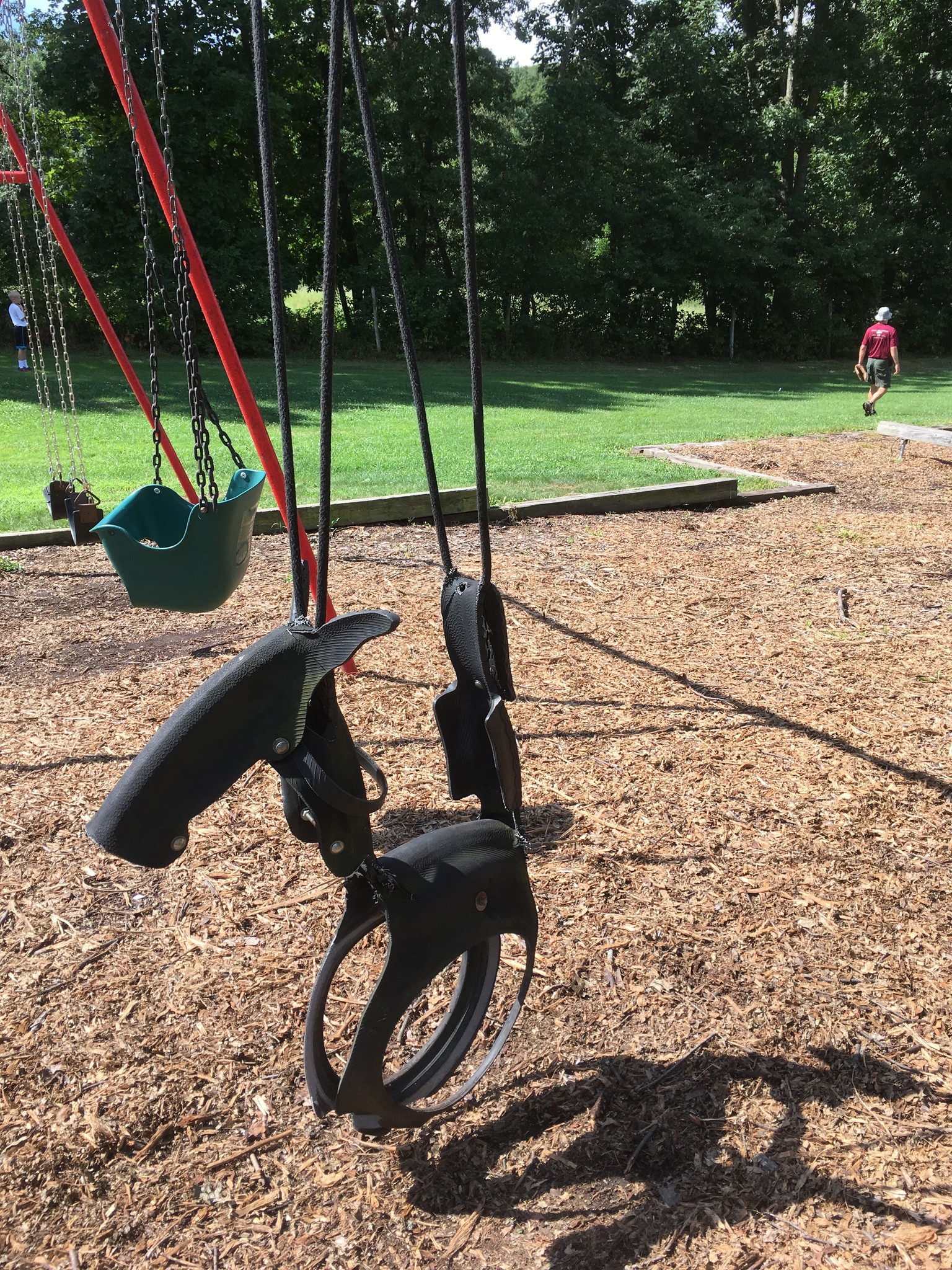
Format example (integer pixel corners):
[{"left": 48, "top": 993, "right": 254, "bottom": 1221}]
[
  {"left": 853, "top": 306, "right": 900, "bottom": 417},
  {"left": 9, "top": 291, "right": 32, "bottom": 372}
]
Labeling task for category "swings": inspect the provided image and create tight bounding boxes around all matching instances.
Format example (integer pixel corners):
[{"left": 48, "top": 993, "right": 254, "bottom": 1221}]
[
  {"left": 3, "top": 0, "right": 104, "bottom": 547},
  {"left": 0, "top": 94, "right": 76, "bottom": 521},
  {"left": 82, "top": 0, "right": 538, "bottom": 1138},
  {"left": 87, "top": 0, "right": 267, "bottom": 614}
]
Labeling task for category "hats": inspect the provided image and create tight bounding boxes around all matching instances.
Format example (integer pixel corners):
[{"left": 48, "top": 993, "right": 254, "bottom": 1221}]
[{"left": 874, "top": 307, "right": 893, "bottom": 321}]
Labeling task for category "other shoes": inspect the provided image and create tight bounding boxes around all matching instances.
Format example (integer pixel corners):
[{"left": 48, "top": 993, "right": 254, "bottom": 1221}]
[
  {"left": 862, "top": 401, "right": 872, "bottom": 417},
  {"left": 872, "top": 409, "right": 876, "bottom": 415},
  {"left": 17, "top": 366, "right": 27, "bottom": 372},
  {"left": 23, "top": 367, "right": 33, "bottom": 372}
]
